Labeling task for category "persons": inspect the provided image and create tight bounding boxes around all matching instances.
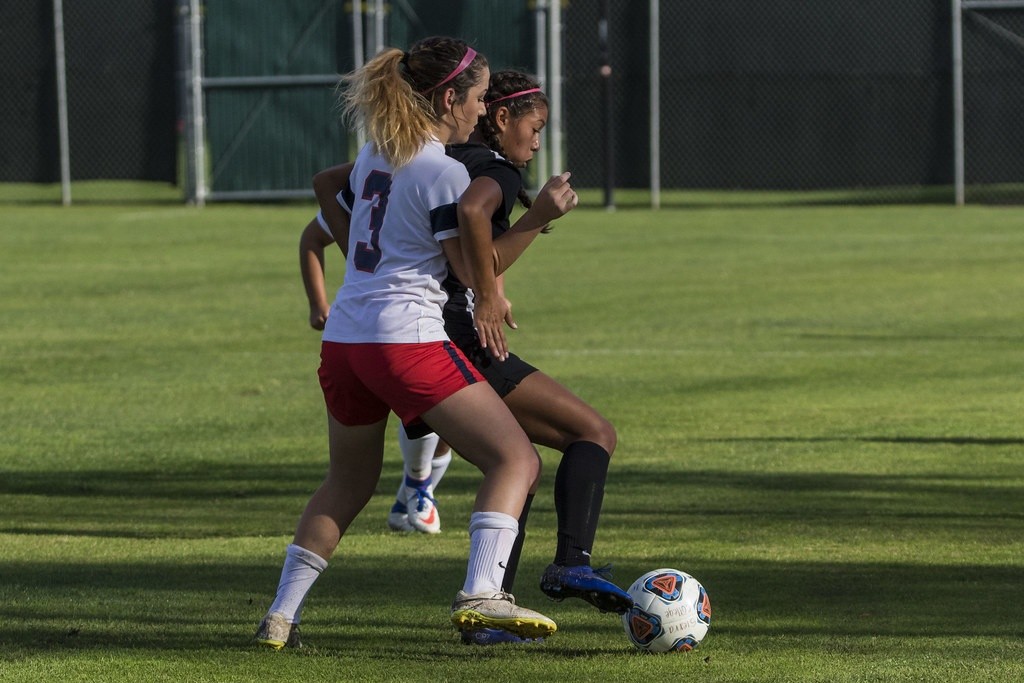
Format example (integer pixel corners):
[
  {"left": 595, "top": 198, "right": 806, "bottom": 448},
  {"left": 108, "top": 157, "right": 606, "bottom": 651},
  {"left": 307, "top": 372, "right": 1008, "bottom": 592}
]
[
  {"left": 313, "top": 72, "right": 634, "bottom": 615},
  {"left": 253, "top": 37, "right": 557, "bottom": 652},
  {"left": 300, "top": 187, "right": 509, "bottom": 536}
]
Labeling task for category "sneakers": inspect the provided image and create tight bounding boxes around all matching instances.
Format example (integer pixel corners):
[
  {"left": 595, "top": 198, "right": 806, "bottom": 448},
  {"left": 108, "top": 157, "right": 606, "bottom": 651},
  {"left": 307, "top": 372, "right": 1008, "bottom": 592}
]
[
  {"left": 449, "top": 589, "right": 558, "bottom": 650},
  {"left": 248, "top": 612, "right": 303, "bottom": 651},
  {"left": 539, "top": 562, "right": 635, "bottom": 617},
  {"left": 386, "top": 466, "right": 442, "bottom": 534}
]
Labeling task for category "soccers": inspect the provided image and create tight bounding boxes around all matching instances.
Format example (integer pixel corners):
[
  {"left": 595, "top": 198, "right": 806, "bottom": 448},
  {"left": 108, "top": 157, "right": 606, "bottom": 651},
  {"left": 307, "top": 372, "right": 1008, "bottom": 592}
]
[{"left": 623, "top": 564, "right": 712, "bottom": 652}]
